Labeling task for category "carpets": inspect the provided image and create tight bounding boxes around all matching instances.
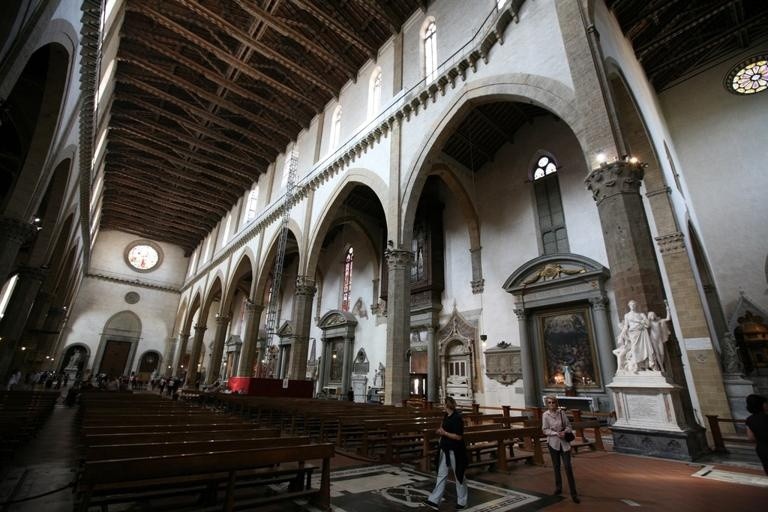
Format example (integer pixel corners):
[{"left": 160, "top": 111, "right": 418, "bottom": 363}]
[{"left": 252, "top": 461, "right": 567, "bottom": 512}]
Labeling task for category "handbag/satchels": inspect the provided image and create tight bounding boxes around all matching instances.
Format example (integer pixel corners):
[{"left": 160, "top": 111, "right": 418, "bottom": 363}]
[{"left": 559, "top": 409, "right": 576, "bottom": 443}]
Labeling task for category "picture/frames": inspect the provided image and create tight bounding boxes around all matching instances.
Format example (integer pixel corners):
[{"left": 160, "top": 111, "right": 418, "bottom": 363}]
[{"left": 535, "top": 306, "right": 602, "bottom": 388}]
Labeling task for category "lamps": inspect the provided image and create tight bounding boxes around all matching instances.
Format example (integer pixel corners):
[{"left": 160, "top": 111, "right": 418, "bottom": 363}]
[{"left": 724, "top": 2, "right": 767, "bottom": 95}]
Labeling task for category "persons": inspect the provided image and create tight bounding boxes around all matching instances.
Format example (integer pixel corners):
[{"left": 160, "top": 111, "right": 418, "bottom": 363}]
[
  {"left": 8, "top": 368, "right": 70, "bottom": 390},
  {"left": 73, "top": 368, "right": 101, "bottom": 396},
  {"left": 101, "top": 368, "right": 181, "bottom": 395},
  {"left": 745, "top": 393, "right": 768, "bottom": 475},
  {"left": 541, "top": 395, "right": 580, "bottom": 504},
  {"left": 421, "top": 397, "right": 470, "bottom": 511},
  {"left": 560, "top": 361, "right": 576, "bottom": 388},
  {"left": 347, "top": 387, "right": 354, "bottom": 402},
  {"left": 612, "top": 297, "right": 671, "bottom": 376}
]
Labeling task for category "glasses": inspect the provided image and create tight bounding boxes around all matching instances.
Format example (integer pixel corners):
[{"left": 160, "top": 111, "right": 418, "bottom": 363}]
[{"left": 546, "top": 401, "right": 556, "bottom": 405}]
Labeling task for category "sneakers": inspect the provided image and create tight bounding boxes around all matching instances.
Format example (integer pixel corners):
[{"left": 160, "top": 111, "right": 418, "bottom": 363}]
[
  {"left": 571, "top": 494, "right": 581, "bottom": 504},
  {"left": 455, "top": 502, "right": 466, "bottom": 510},
  {"left": 553, "top": 488, "right": 564, "bottom": 496},
  {"left": 421, "top": 500, "right": 439, "bottom": 511}
]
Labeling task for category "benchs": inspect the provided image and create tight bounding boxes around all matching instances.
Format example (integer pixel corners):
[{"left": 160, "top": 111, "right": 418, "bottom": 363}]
[
  {"left": 200, "top": 390, "right": 544, "bottom": 472},
  {"left": 2, "top": 388, "right": 60, "bottom": 465},
  {"left": 78, "top": 389, "right": 335, "bottom": 512}
]
